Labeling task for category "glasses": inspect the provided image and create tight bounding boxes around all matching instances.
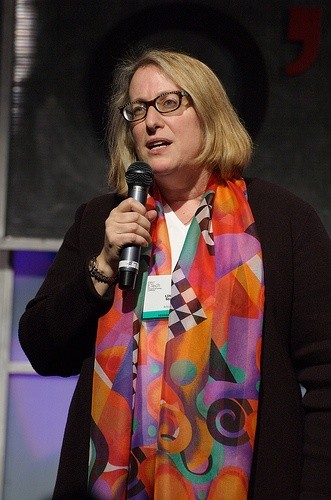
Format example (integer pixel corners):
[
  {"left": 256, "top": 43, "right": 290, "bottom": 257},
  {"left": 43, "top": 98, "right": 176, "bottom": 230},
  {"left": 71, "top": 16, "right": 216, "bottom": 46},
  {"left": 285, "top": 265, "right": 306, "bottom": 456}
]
[{"left": 118, "top": 89, "right": 189, "bottom": 122}]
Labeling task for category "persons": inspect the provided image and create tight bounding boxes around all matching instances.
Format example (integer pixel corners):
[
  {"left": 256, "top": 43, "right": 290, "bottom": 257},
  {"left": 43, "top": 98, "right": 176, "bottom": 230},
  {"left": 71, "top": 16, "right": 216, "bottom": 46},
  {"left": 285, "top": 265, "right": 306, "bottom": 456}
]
[{"left": 18, "top": 49, "right": 331, "bottom": 500}]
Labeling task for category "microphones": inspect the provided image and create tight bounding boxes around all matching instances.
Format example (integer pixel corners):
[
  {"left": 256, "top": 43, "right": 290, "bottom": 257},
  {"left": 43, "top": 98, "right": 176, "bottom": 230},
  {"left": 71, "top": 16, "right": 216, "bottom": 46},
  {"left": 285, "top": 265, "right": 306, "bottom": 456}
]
[{"left": 118, "top": 161, "right": 154, "bottom": 291}]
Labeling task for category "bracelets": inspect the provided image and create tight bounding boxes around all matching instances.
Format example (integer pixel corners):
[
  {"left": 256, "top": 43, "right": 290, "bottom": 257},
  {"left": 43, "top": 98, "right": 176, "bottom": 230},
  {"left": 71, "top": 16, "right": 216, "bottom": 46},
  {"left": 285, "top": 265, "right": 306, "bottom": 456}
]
[{"left": 88, "top": 256, "right": 118, "bottom": 285}]
